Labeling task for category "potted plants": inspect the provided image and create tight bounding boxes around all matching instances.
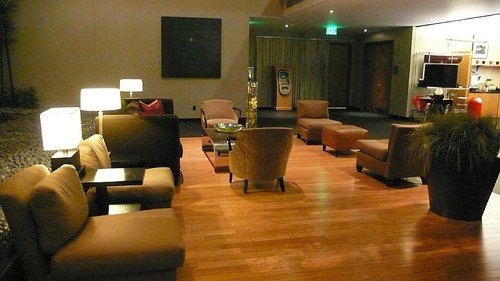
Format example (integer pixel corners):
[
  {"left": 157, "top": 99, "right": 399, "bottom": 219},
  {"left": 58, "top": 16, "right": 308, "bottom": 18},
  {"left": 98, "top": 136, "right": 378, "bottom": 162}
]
[{"left": 400, "top": 104, "right": 500, "bottom": 220}]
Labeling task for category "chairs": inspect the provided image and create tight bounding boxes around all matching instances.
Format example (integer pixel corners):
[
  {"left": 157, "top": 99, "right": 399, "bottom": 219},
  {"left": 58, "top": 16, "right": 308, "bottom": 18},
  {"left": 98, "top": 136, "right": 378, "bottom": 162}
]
[
  {"left": 228, "top": 128, "right": 293, "bottom": 193},
  {"left": 201, "top": 99, "right": 242, "bottom": 128}
]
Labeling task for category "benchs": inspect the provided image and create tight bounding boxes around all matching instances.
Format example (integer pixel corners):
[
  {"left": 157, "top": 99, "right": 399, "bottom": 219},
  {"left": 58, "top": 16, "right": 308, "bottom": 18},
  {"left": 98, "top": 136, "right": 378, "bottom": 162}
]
[{"left": 322, "top": 125, "right": 368, "bottom": 157}]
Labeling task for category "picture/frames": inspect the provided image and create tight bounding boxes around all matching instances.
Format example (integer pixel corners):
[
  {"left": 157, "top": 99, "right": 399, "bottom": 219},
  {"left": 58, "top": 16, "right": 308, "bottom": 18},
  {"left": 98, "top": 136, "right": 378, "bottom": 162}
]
[{"left": 472, "top": 41, "right": 488, "bottom": 59}]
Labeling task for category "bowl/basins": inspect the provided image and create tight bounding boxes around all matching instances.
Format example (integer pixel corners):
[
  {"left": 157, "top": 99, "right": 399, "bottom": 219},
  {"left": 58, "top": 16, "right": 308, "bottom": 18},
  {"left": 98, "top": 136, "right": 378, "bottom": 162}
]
[{"left": 214, "top": 123, "right": 244, "bottom": 133}]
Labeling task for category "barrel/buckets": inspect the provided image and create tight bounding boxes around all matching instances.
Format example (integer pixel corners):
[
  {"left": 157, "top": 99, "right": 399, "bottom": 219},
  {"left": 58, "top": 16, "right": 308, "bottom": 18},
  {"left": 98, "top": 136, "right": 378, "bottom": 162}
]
[{"left": 469, "top": 72, "right": 481, "bottom": 89}]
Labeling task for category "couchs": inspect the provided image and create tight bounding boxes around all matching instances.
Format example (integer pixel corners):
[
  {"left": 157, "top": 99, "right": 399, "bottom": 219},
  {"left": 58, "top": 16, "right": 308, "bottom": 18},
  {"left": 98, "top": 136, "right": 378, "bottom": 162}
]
[
  {"left": 96, "top": 115, "right": 181, "bottom": 187},
  {"left": 123, "top": 98, "right": 174, "bottom": 114},
  {"left": 357, "top": 124, "right": 429, "bottom": 186},
  {"left": 0, "top": 164, "right": 186, "bottom": 281},
  {"left": 297, "top": 100, "right": 343, "bottom": 145},
  {"left": 88, "top": 167, "right": 175, "bottom": 210}
]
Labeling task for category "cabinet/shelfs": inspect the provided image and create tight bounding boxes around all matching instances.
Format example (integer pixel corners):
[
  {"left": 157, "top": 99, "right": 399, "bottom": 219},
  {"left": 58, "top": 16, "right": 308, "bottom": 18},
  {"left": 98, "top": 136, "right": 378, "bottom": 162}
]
[{"left": 429, "top": 87, "right": 469, "bottom": 114}]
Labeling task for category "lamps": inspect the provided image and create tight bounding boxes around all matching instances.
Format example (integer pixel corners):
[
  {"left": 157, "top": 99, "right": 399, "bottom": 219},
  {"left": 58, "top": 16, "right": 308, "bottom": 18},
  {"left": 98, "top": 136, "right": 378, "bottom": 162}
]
[
  {"left": 120, "top": 78, "right": 144, "bottom": 98},
  {"left": 80, "top": 87, "right": 121, "bottom": 156},
  {"left": 40, "top": 106, "right": 85, "bottom": 177}
]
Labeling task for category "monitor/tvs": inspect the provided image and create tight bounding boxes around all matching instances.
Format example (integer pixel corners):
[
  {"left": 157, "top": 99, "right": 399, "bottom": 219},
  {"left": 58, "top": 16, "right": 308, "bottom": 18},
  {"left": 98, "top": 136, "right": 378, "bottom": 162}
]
[{"left": 423, "top": 63, "right": 459, "bottom": 88}]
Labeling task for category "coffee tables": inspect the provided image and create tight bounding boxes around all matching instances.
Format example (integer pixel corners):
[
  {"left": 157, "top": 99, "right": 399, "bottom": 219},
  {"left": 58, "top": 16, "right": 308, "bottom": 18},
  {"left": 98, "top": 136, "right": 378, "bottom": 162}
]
[
  {"left": 202, "top": 128, "right": 235, "bottom": 173},
  {"left": 81, "top": 165, "right": 146, "bottom": 216}
]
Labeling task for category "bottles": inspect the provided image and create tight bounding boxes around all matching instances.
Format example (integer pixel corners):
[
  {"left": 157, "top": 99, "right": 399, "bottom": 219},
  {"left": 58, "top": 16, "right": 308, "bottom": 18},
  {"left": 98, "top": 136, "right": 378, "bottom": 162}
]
[{"left": 484, "top": 79, "right": 494, "bottom": 91}]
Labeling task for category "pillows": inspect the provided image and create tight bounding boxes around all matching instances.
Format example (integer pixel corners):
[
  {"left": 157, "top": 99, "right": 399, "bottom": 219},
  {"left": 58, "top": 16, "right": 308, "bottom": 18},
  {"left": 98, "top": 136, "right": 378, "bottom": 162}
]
[
  {"left": 79, "top": 134, "right": 111, "bottom": 174},
  {"left": 31, "top": 165, "right": 89, "bottom": 254},
  {"left": 139, "top": 100, "right": 164, "bottom": 114}
]
